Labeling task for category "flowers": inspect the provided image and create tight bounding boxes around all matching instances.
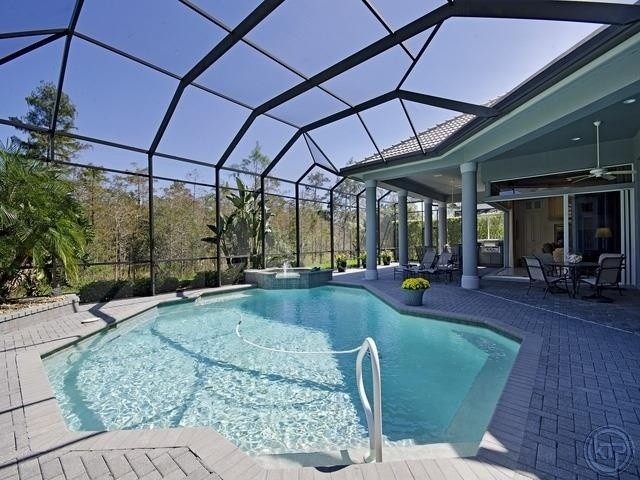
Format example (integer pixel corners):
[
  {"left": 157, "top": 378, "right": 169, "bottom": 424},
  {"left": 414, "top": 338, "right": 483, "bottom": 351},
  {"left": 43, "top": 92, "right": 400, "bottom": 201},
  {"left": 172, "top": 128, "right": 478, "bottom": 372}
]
[{"left": 400, "top": 277, "right": 431, "bottom": 290}]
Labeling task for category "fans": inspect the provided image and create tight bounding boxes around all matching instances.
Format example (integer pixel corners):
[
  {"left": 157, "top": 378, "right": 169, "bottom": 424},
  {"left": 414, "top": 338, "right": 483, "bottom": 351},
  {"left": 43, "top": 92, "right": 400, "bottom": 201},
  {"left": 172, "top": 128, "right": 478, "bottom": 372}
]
[
  {"left": 566, "top": 122, "right": 637, "bottom": 185},
  {"left": 439, "top": 176, "right": 462, "bottom": 210}
]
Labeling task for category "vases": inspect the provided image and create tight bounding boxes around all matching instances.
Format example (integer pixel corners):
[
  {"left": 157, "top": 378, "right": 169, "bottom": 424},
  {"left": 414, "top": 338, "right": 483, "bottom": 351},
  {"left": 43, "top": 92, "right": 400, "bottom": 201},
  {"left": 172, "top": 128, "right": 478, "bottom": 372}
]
[{"left": 403, "top": 288, "right": 426, "bottom": 306}]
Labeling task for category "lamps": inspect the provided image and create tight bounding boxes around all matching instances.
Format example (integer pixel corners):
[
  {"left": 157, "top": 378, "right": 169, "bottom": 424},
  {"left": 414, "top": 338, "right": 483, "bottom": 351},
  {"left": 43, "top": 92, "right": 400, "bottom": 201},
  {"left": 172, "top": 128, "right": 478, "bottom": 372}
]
[{"left": 594, "top": 227, "right": 612, "bottom": 250}]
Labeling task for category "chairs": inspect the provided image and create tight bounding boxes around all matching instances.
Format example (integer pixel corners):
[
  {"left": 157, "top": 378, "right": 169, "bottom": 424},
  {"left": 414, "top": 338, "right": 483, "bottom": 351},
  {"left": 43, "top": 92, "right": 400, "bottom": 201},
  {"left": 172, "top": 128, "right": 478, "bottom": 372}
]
[
  {"left": 518, "top": 247, "right": 626, "bottom": 299},
  {"left": 391, "top": 241, "right": 464, "bottom": 287}
]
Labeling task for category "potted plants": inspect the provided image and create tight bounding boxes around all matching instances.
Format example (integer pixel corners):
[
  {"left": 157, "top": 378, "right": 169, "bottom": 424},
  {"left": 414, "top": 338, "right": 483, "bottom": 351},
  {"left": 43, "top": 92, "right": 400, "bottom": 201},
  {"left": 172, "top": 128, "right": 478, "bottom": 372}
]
[
  {"left": 359, "top": 251, "right": 367, "bottom": 268},
  {"left": 380, "top": 249, "right": 391, "bottom": 265},
  {"left": 335, "top": 253, "right": 349, "bottom": 273}
]
